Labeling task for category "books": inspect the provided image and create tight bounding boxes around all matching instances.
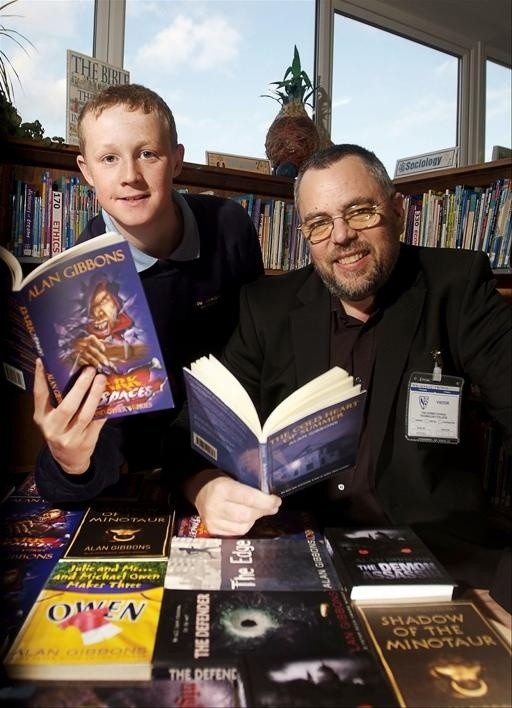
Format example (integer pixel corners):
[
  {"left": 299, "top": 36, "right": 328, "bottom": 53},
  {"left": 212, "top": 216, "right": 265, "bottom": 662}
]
[
  {"left": 27, "top": 663, "right": 245, "bottom": 708},
  {"left": 1, "top": 557, "right": 167, "bottom": 680},
  {"left": 232, "top": 193, "right": 311, "bottom": 272},
  {"left": 165, "top": 534, "right": 343, "bottom": 591},
  {"left": 0, "top": 498, "right": 87, "bottom": 558},
  {"left": 400, "top": 174, "right": 511, "bottom": 268},
  {"left": 183, "top": 354, "right": 368, "bottom": 501},
  {"left": 355, "top": 599, "right": 511, "bottom": 707},
  {"left": 248, "top": 659, "right": 396, "bottom": 707},
  {"left": 0, "top": 232, "right": 176, "bottom": 424},
  {"left": 151, "top": 590, "right": 372, "bottom": 678},
  {"left": 320, "top": 524, "right": 461, "bottom": 600},
  {"left": 0, "top": 167, "right": 102, "bottom": 258},
  {"left": 62, "top": 509, "right": 174, "bottom": 557}
]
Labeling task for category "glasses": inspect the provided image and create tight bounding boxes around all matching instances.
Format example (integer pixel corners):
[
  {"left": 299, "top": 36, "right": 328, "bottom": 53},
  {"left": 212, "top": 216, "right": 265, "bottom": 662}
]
[{"left": 295, "top": 194, "right": 394, "bottom": 245}]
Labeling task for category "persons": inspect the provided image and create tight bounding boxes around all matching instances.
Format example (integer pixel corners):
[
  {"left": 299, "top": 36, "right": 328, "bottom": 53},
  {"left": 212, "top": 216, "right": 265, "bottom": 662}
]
[
  {"left": 160, "top": 144, "right": 511, "bottom": 630},
  {"left": 32, "top": 84, "right": 265, "bottom": 509}
]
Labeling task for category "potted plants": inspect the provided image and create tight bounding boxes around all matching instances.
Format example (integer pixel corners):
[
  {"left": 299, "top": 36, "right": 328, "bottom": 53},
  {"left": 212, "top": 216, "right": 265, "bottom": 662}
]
[{"left": 264, "top": 44, "right": 322, "bottom": 175}]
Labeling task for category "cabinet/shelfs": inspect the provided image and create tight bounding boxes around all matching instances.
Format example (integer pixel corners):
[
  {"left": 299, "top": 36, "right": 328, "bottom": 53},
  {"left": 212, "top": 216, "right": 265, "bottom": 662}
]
[{"left": 0, "top": 137, "right": 511, "bottom": 299}]
